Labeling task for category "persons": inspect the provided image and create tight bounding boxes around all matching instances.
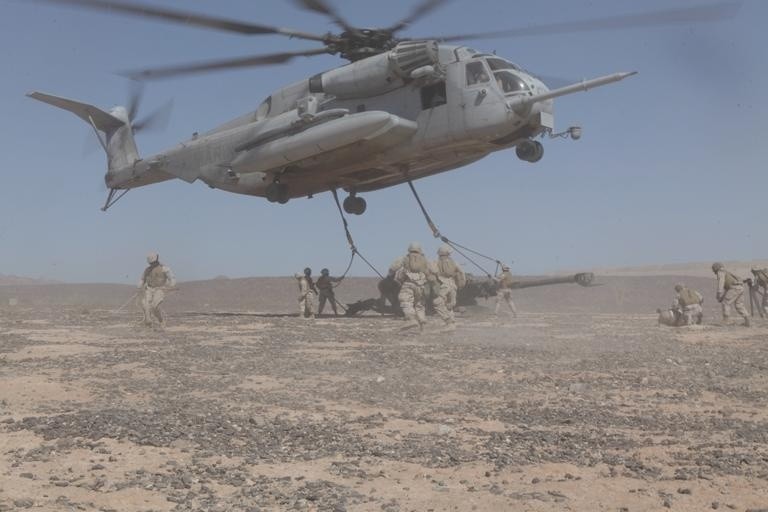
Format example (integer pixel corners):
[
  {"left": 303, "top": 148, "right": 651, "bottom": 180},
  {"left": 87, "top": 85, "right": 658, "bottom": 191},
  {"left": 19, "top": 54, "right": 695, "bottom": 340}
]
[
  {"left": 489, "top": 260, "right": 517, "bottom": 317},
  {"left": 316, "top": 268, "right": 344, "bottom": 317},
  {"left": 429, "top": 243, "right": 465, "bottom": 332},
  {"left": 295, "top": 268, "right": 318, "bottom": 317},
  {"left": 712, "top": 262, "right": 751, "bottom": 327},
  {"left": 139, "top": 252, "right": 175, "bottom": 328},
  {"left": 390, "top": 243, "right": 433, "bottom": 330},
  {"left": 656, "top": 308, "right": 682, "bottom": 325},
  {"left": 752, "top": 264, "right": 768, "bottom": 319},
  {"left": 674, "top": 285, "right": 704, "bottom": 326}
]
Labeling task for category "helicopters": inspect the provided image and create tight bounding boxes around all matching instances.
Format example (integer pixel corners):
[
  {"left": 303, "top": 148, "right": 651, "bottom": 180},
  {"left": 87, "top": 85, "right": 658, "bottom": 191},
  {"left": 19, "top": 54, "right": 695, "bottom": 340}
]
[{"left": 25, "top": 0, "right": 744, "bottom": 216}]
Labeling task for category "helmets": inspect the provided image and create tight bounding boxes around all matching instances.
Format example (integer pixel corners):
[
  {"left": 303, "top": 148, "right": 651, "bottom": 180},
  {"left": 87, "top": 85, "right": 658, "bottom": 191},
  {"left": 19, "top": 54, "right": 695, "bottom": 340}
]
[
  {"left": 438, "top": 247, "right": 449, "bottom": 256},
  {"left": 409, "top": 242, "right": 420, "bottom": 253},
  {"left": 147, "top": 253, "right": 158, "bottom": 264},
  {"left": 502, "top": 265, "right": 509, "bottom": 271}
]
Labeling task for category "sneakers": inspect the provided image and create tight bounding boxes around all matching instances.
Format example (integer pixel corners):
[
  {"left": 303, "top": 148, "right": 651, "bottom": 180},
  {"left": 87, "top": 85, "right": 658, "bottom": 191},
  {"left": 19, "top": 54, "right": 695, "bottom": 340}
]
[{"left": 399, "top": 317, "right": 457, "bottom": 332}]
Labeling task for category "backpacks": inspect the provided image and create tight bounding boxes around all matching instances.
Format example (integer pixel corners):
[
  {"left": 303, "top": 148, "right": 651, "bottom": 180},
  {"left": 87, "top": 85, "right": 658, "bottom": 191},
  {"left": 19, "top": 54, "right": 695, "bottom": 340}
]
[
  {"left": 408, "top": 254, "right": 425, "bottom": 273},
  {"left": 438, "top": 257, "right": 456, "bottom": 277}
]
